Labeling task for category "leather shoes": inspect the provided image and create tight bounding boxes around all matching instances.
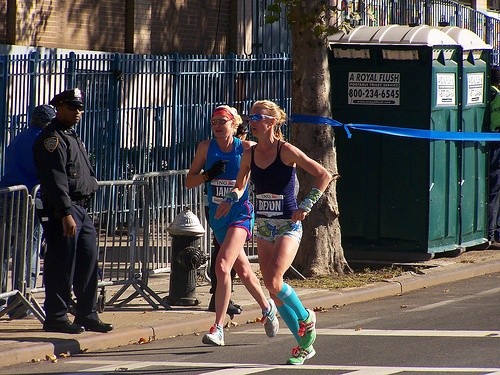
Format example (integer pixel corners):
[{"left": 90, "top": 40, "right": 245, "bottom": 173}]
[
  {"left": 73, "top": 311, "right": 113, "bottom": 331},
  {"left": 43, "top": 318, "right": 85, "bottom": 333}
]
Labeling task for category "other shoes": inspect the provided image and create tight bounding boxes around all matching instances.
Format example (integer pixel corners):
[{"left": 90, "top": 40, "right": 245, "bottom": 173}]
[{"left": 208, "top": 295, "right": 242, "bottom": 314}]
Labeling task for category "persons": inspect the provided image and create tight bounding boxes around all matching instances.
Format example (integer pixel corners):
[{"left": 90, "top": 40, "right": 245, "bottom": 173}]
[
  {"left": 0, "top": 105, "right": 56, "bottom": 319},
  {"left": 213, "top": 100, "right": 332, "bottom": 365},
  {"left": 32, "top": 88, "right": 114, "bottom": 334},
  {"left": 184, "top": 105, "right": 279, "bottom": 346},
  {"left": 487, "top": 68, "right": 500, "bottom": 250}
]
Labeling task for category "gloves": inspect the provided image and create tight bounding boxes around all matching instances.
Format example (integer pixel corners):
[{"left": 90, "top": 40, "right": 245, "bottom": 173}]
[{"left": 208, "top": 159, "right": 229, "bottom": 178}]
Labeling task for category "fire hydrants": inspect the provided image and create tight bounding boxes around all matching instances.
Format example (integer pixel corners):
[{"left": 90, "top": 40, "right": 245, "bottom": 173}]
[{"left": 163, "top": 207, "right": 207, "bottom": 306}]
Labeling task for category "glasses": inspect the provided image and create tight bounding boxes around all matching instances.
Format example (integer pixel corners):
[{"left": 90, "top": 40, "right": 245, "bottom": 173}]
[
  {"left": 210, "top": 119, "right": 230, "bottom": 126},
  {"left": 66, "top": 105, "right": 85, "bottom": 111},
  {"left": 235, "top": 128, "right": 247, "bottom": 135},
  {"left": 249, "top": 114, "right": 274, "bottom": 122}
]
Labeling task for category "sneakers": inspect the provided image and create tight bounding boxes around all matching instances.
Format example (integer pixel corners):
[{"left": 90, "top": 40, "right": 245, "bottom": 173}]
[
  {"left": 202, "top": 323, "right": 225, "bottom": 345},
  {"left": 297, "top": 308, "right": 316, "bottom": 350},
  {"left": 287, "top": 344, "right": 316, "bottom": 364},
  {"left": 261, "top": 299, "right": 279, "bottom": 337}
]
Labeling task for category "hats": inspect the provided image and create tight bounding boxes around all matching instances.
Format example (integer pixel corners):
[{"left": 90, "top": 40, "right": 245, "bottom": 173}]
[
  {"left": 50, "top": 88, "right": 84, "bottom": 107},
  {"left": 31, "top": 105, "right": 57, "bottom": 125}
]
